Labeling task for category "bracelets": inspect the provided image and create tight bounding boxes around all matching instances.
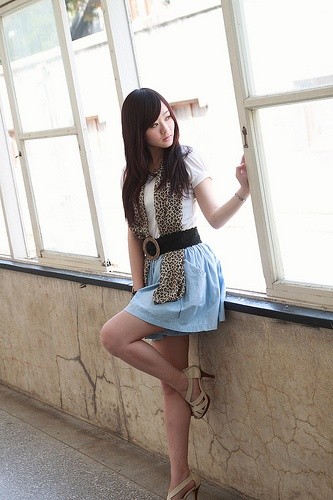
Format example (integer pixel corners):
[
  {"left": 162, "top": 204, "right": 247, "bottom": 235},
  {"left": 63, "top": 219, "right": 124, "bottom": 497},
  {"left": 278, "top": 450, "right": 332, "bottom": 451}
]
[{"left": 234, "top": 193, "right": 247, "bottom": 202}]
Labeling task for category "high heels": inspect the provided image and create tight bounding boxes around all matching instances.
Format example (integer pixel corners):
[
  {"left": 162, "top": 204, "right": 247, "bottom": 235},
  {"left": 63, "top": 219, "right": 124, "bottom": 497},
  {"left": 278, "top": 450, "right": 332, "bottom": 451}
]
[
  {"left": 182, "top": 365, "right": 215, "bottom": 419},
  {"left": 166, "top": 470, "right": 201, "bottom": 500}
]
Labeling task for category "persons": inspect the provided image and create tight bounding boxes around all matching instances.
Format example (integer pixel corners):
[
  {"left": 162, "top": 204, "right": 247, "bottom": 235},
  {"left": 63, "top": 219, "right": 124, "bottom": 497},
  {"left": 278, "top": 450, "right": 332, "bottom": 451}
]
[{"left": 100, "top": 87, "right": 251, "bottom": 500}]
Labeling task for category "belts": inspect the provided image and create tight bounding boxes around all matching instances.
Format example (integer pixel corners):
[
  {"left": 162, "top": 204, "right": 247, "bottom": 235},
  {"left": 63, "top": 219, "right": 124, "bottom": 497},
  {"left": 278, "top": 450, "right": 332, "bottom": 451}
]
[{"left": 143, "top": 226, "right": 202, "bottom": 261}]
